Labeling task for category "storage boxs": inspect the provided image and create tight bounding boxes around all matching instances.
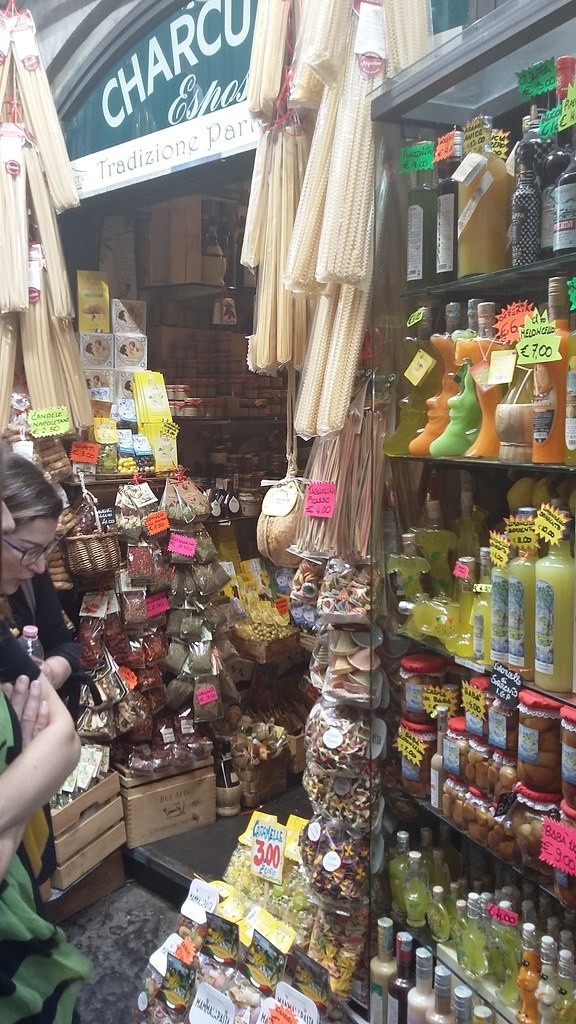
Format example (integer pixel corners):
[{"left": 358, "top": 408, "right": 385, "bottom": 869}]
[{"left": 50, "top": 669, "right": 317, "bottom": 892}]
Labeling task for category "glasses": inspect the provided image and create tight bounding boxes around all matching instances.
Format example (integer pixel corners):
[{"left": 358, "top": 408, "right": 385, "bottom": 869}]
[{"left": 2, "top": 536, "right": 60, "bottom": 567}]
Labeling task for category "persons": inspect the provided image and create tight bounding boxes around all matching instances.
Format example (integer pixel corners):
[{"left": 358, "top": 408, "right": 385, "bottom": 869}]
[
  {"left": 1, "top": 453, "right": 84, "bottom": 923},
  {"left": 0, "top": 437, "right": 95, "bottom": 1024}
]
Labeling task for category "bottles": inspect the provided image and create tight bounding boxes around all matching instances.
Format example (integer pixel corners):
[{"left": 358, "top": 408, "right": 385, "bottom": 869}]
[
  {"left": 339, "top": 51, "right": 576, "bottom": 1023},
  {"left": 164, "top": 347, "right": 292, "bottom": 527}
]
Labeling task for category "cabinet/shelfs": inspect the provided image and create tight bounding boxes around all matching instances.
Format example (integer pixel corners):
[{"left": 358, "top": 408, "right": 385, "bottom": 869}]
[
  {"left": 172, "top": 415, "right": 287, "bottom": 524},
  {"left": 364, "top": 0, "right": 575, "bottom": 1024}
]
